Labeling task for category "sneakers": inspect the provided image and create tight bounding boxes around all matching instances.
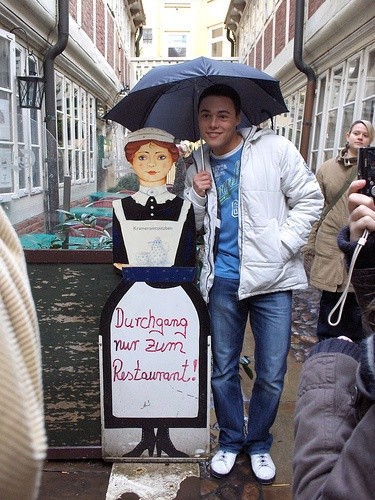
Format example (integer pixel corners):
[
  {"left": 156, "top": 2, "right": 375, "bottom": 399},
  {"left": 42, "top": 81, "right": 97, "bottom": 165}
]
[
  {"left": 250, "top": 450, "right": 276, "bottom": 484},
  {"left": 209, "top": 451, "right": 239, "bottom": 479}
]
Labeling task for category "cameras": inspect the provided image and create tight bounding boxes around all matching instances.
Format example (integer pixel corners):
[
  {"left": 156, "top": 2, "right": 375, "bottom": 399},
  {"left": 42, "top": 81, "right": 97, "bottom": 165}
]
[{"left": 356, "top": 147, "right": 375, "bottom": 204}]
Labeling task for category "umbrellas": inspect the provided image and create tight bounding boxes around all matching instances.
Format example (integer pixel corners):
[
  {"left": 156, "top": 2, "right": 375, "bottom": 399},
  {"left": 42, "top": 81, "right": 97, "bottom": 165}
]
[{"left": 103, "top": 56, "right": 290, "bottom": 171}]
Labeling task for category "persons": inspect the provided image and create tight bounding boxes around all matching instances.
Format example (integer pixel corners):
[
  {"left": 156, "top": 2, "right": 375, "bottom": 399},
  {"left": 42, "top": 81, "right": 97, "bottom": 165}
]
[
  {"left": 183, "top": 84, "right": 325, "bottom": 484},
  {"left": 307, "top": 120, "right": 375, "bottom": 341},
  {"left": 291, "top": 178, "right": 375, "bottom": 500},
  {"left": 0, "top": 205, "right": 47, "bottom": 500}
]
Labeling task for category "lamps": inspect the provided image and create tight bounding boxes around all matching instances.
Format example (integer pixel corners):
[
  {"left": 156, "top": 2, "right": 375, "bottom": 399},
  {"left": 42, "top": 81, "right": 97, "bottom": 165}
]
[
  {"left": 10, "top": 27, "right": 45, "bottom": 110},
  {"left": 113, "top": 85, "right": 130, "bottom": 106}
]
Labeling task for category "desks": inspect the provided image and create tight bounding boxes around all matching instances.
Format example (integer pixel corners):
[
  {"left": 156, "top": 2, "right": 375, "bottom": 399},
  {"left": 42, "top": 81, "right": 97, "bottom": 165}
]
[
  {"left": 88, "top": 192, "right": 128, "bottom": 204},
  {"left": 70, "top": 206, "right": 113, "bottom": 218},
  {"left": 19, "top": 233, "right": 100, "bottom": 249}
]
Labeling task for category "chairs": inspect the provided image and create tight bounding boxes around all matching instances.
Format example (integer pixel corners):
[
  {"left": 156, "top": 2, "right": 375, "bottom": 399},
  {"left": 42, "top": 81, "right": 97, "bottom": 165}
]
[
  {"left": 68, "top": 217, "right": 113, "bottom": 238},
  {"left": 86, "top": 197, "right": 122, "bottom": 208}
]
